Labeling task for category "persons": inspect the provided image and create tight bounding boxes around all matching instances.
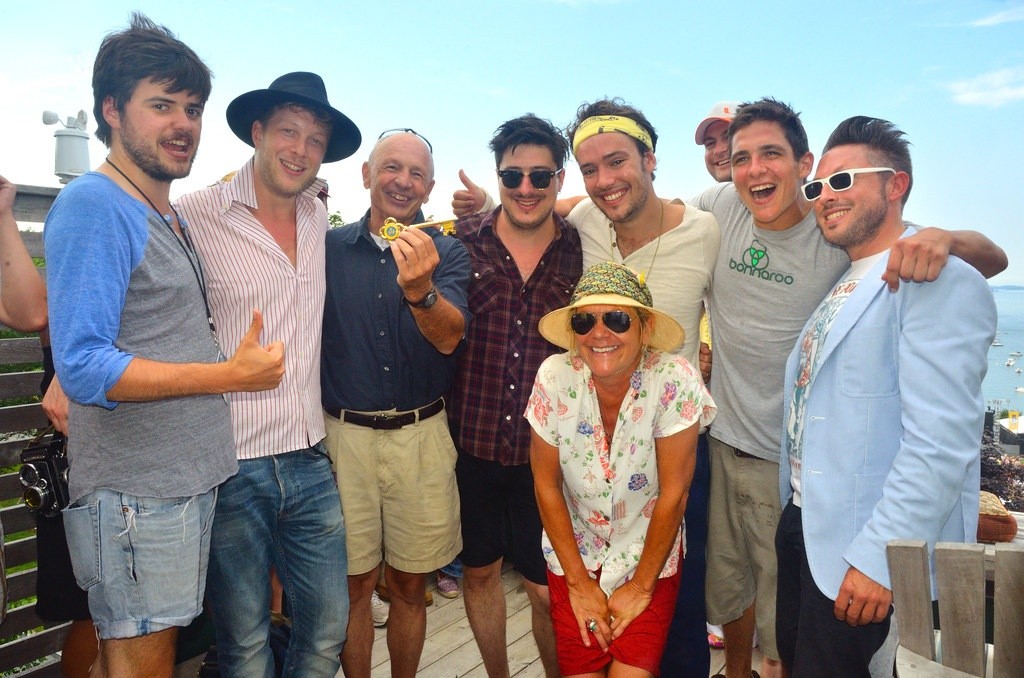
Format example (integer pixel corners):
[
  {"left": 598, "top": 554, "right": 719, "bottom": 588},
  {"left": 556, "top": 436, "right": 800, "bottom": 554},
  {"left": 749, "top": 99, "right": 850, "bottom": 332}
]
[
  {"left": 325, "top": 128, "right": 474, "bottom": 678},
  {"left": 42, "top": 12, "right": 286, "bottom": 678},
  {"left": 563, "top": 100, "right": 721, "bottom": 678},
  {"left": 38, "top": 326, "right": 99, "bottom": 678},
  {"left": 44, "top": 72, "right": 362, "bottom": 678},
  {"left": 694, "top": 102, "right": 750, "bottom": 646},
  {"left": 444, "top": 117, "right": 713, "bottom": 678},
  {"left": 0, "top": 174, "right": 49, "bottom": 332},
  {"left": 273, "top": 558, "right": 462, "bottom": 627},
  {"left": 453, "top": 96, "right": 1008, "bottom": 678},
  {"left": 773, "top": 116, "right": 997, "bottom": 678},
  {"left": 523, "top": 262, "right": 719, "bottom": 678}
]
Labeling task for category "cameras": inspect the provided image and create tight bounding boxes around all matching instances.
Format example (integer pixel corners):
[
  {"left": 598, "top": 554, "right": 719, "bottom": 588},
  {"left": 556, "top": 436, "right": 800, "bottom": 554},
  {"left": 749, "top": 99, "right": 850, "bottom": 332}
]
[{"left": 18, "top": 438, "right": 71, "bottom": 524}]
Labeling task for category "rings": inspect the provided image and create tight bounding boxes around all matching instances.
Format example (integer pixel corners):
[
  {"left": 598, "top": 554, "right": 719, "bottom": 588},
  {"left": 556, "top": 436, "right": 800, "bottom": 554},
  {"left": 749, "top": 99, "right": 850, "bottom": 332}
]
[
  {"left": 707, "top": 373, "right": 709, "bottom": 377},
  {"left": 587, "top": 618, "right": 596, "bottom": 631},
  {"left": 610, "top": 615, "right": 615, "bottom": 622}
]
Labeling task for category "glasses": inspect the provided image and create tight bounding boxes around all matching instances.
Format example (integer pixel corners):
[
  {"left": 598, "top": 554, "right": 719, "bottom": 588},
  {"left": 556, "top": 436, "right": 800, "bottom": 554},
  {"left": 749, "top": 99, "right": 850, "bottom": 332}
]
[
  {"left": 568, "top": 310, "right": 639, "bottom": 335},
  {"left": 496, "top": 167, "right": 563, "bottom": 189},
  {"left": 378, "top": 128, "right": 433, "bottom": 156},
  {"left": 801, "top": 167, "right": 897, "bottom": 201}
]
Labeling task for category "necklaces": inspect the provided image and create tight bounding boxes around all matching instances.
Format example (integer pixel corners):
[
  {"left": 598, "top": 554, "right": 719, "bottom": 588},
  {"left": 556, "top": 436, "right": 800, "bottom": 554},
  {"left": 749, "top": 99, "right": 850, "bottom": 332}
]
[{"left": 611, "top": 198, "right": 663, "bottom": 282}]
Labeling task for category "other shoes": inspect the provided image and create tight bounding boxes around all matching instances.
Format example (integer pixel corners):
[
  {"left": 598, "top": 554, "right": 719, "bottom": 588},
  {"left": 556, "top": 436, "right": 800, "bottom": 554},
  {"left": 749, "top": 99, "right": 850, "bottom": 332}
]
[
  {"left": 374, "top": 585, "right": 433, "bottom": 607},
  {"left": 435, "top": 569, "right": 460, "bottom": 598},
  {"left": 709, "top": 632, "right": 727, "bottom": 647}
]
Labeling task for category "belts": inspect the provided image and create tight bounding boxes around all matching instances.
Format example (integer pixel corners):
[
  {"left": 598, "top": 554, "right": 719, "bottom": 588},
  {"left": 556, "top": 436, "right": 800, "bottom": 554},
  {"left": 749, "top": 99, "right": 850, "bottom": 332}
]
[{"left": 322, "top": 399, "right": 444, "bottom": 430}]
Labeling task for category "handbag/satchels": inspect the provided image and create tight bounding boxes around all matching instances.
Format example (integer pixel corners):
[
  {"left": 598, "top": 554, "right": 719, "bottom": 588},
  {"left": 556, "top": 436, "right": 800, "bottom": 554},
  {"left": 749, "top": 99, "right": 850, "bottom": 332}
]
[{"left": 978, "top": 491, "right": 1017, "bottom": 544}]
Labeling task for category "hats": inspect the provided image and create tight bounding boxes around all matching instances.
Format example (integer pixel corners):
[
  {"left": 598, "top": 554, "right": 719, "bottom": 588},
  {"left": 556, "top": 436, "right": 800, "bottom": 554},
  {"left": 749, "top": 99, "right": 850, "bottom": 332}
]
[
  {"left": 226, "top": 72, "right": 362, "bottom": 163},
  {"left": 538, "top": 261, "right": 686, "bottom": 353},
  {"left": 695, "top": 101, "right": 744, "bottom": 145}
]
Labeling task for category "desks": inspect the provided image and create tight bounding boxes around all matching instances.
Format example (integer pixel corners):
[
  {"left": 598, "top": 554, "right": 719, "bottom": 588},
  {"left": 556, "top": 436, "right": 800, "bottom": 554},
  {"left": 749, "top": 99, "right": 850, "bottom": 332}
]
[{"left": 984, "top": 511, "right": 1024, "bottom": 582}]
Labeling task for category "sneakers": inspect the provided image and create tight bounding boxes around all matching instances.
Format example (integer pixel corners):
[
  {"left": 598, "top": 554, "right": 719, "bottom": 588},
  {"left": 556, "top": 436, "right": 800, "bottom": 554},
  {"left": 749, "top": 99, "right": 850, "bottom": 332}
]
[{"left": 370, "top": 589, "right": 390, "bottom": 627}]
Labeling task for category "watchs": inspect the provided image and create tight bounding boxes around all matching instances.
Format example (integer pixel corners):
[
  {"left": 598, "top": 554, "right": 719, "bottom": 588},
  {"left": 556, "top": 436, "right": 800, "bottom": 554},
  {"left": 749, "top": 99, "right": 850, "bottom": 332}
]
[{"left": 400, "top": 286, "right": 438, "bottom": 309}]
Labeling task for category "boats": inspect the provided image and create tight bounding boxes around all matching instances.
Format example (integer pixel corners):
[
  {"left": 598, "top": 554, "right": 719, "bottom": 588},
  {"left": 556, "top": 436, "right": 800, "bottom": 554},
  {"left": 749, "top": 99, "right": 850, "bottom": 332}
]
[
  {"left": 1010, "top": 352, "right": 1023, "bottom": 357},
  {"left": 1016, "top": 369, "right": 1022, "bottom": 372},
  {"left": 1014, "top": 388, "right": 1024, "bottom": 393},
  {"left": 993, "top": 339, "right": 1004, "bottom": 346},
  {"left": 1007, "top": 359, "right": 1014, "bottom": 366}
]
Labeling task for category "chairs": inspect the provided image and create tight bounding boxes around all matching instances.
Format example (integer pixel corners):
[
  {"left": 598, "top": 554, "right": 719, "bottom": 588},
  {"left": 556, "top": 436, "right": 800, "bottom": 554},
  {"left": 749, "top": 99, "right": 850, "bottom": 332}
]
[{"left": 885, "top": 542, "right": 1024, "bottom": 678}]
